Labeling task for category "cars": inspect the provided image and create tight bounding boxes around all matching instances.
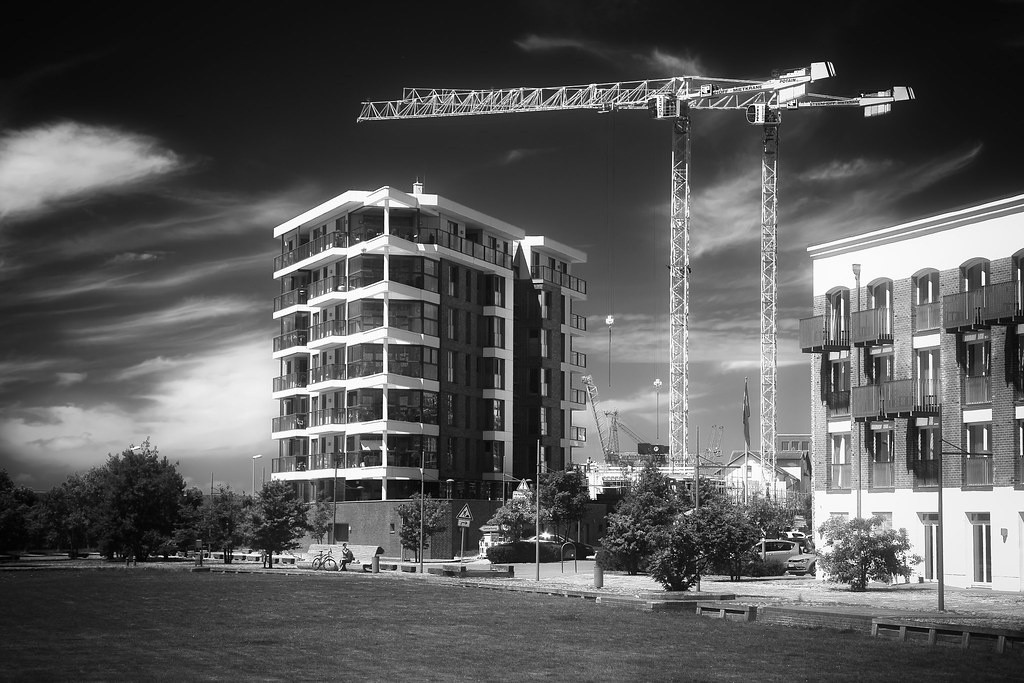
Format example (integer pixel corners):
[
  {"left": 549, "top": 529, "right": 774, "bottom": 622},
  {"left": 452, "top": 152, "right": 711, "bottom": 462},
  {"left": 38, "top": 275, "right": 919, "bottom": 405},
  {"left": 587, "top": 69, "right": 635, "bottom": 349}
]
[{"left": 528, "top": 532, "right": 596, "bottom": 561}]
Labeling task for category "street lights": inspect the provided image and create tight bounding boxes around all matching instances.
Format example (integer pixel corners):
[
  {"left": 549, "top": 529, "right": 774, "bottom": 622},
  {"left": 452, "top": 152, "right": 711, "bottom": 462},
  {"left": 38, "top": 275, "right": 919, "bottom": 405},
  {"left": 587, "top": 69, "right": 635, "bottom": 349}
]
[
  {"left": 419, "top": 451, "right": 454, "bottom": 573},
  {"left": 332, "top": 461, "right": 364, "bottom": 544},
  {"left": 252, "top": 454, "right": 263, "bottom": 499},
  {"left": 502, "top": 455, "right": 533, "bottom": 507},
  {"left": 937, "top": 402, "right": 994, "bottom": 612}
]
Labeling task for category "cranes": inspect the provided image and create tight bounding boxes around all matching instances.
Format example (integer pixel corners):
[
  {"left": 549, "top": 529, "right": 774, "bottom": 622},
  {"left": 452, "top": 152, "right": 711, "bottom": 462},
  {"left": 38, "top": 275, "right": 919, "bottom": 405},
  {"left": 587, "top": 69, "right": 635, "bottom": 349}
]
[{"left": 354, "top": 60, "right": 916, "bottom": 509}]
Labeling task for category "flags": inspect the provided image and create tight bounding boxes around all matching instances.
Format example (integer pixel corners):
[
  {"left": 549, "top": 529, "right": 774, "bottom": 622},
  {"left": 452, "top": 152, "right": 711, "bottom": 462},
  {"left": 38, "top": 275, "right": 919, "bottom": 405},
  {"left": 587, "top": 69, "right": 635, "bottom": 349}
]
[{"left": 743, "top": 383, "right": 750, "bottom": 449}]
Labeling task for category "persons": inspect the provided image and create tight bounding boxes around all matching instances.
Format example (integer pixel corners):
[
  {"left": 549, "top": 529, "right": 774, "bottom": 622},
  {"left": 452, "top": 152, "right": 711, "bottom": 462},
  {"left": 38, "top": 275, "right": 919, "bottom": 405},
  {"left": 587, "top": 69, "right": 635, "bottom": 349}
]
[{"left": 340, "top": 543, "right": 351, "bottom": 571}]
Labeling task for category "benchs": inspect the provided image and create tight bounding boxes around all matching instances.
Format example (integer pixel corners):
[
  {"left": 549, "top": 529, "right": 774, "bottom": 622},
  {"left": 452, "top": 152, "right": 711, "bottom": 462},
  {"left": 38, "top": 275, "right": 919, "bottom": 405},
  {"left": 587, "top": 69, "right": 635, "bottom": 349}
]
[{"left": 297, "top": 543, "right": 385, "bottom": 572}]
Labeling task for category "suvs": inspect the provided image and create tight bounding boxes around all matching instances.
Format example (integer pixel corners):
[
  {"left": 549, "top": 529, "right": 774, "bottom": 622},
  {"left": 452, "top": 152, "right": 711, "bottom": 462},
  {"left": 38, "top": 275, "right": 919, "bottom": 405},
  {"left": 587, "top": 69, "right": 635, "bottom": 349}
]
[{"left": 742, "top": 532, "right": 815, "bottom": 576}]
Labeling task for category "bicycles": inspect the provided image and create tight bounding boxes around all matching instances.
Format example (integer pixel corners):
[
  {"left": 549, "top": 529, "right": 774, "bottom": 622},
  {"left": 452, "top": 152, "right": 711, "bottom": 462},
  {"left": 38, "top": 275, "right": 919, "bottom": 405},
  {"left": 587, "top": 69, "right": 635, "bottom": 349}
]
[{"left": 311, "top": 549, "right": 336, "bottom": 571}]
